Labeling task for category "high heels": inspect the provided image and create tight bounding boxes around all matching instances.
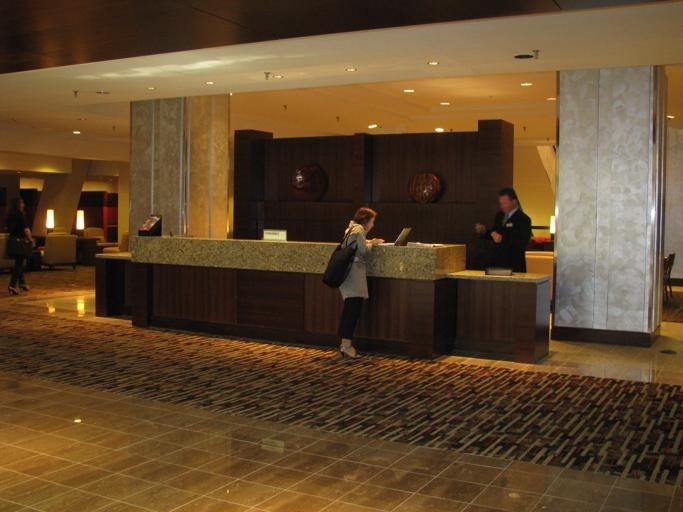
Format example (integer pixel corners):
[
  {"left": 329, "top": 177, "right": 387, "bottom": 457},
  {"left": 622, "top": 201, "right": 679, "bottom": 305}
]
[
  {"left": 8, "top": 285, "right": 19, "bottom": 296},
  {"left": 19, "top": 283, "right": 30, "bottom": 292},
  {"left": 340, "top": 345, "right": 362, "bottom": 359}
]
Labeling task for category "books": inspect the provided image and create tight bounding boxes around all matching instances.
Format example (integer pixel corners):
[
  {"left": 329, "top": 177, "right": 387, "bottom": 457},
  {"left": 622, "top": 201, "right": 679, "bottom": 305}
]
[
  {"left": 485, "top": 267, "right": 514, "bottom": 276},
  {"left": 407, "top": 241, "right": 443, "bottom": 247}
]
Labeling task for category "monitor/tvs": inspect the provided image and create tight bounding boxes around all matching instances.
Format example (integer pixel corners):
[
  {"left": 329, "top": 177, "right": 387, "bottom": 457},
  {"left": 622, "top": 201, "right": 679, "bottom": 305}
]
[{"left": 262, "top": 229, "right": 287, "bottom": 240}]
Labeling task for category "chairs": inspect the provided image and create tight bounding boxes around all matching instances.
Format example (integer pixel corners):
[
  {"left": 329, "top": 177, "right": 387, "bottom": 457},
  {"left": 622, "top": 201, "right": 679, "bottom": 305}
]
[
  {"left": 83, "top": 227, "right": 118, "bottom": 253},
  {"left": 663, "top": 256, "right": 669, "bottom": 297},
  {"left": 40, "top": 234, "right": 77, "bottom": 270},
  {"left": 0, "top": 233, "right": 27, "bottom": 274},
  {"left": 668, "top": 253, "right": 675, "bottom": 297}
]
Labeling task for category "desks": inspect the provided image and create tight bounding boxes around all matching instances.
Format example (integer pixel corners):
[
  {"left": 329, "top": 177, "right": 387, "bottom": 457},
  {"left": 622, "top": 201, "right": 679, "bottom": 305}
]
[{"left": 76, "top": 237, "right": 101, "bottom": 265}]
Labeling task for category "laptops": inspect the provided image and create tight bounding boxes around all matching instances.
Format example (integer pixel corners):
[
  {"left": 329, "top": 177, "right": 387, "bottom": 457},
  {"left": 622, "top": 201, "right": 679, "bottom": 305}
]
[{"left": 379, "top": 227, "right": 411, "bottom": 245}]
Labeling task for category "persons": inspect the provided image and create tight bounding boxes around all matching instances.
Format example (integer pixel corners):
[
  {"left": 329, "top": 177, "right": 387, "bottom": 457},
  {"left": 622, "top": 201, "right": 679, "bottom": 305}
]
[
  {"left": 473, "top": 187, "right": 534, "bottom": 272},
  {"left": 336, "top": 208, "right": 385, "bottom": 358},
  {"left": 6, "top": 196, "right": 35, "bottom": 296}
]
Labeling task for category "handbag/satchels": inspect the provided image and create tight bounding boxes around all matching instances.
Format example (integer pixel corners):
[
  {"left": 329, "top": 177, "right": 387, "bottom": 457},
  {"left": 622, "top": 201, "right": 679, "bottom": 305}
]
[
  {"left": 6, "top": 236, "right": 32, "bottom": 257},
  {"left": 322, "top": 225, "right": 357, "bottom": 289},
  {"left": 470, "top": 232, "right": 505, "bottom": 265}
]
